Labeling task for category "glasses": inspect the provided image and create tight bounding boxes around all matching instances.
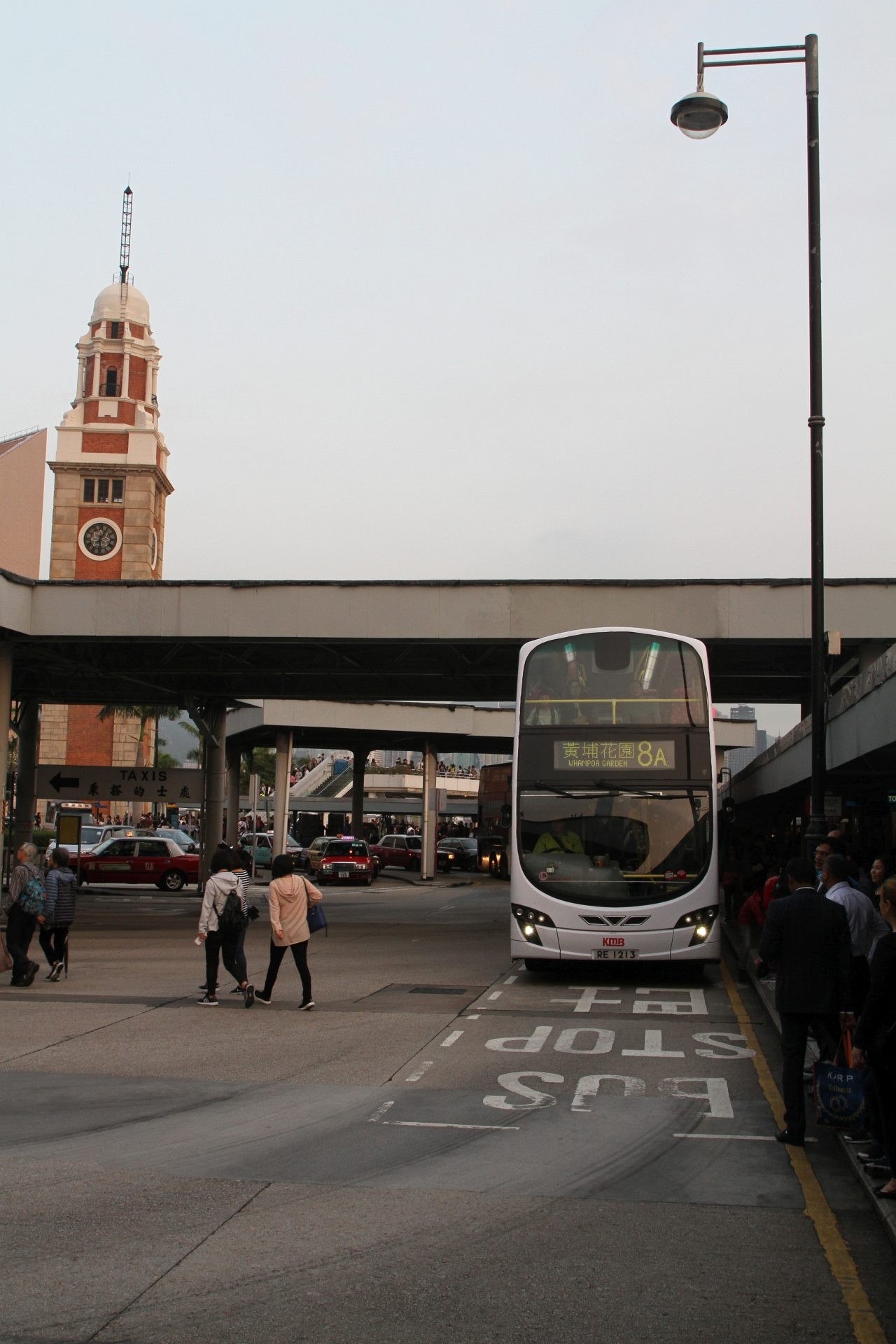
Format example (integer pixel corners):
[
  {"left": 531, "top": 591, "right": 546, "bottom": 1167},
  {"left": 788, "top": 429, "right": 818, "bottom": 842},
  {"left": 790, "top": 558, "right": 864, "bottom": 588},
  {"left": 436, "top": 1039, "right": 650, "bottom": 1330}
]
[{"left": 813, "top": 851, "right": 829, "bottom": 857}]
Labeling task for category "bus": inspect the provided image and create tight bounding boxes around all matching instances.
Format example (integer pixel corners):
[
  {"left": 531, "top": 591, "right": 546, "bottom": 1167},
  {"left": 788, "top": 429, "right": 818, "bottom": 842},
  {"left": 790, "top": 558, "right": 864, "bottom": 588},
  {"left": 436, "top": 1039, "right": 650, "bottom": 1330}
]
[
  {"left": 509, "top": 626, "right": 728, "bottom": 965},
  {"left": 476, "top": 762, "right": 511, "bottom": 880}
]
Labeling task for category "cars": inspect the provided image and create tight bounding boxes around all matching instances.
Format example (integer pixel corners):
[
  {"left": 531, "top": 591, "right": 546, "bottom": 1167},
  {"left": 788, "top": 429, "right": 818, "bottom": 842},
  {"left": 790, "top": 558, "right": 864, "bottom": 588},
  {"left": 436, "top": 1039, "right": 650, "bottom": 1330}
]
[
  {"left": 45, "top": 823, "right": 202, "bottom": 857},
  {"left": 436, "top": 838, "right": 479, "bottom": 869},
  {"left": 318, "top": 840, "right": 373, "bottom": 886},
  {"left": 240, "top": 830, "right": 303, "bottom": 868},
  {"left": 307, "top": 836, "right": 383, "bottom": 873},
  {"left": 68, "top": 836, "right": 203, "bottom": 891},
  {"left": 374, "top": 834, "right": 458, "bottom": 873}
]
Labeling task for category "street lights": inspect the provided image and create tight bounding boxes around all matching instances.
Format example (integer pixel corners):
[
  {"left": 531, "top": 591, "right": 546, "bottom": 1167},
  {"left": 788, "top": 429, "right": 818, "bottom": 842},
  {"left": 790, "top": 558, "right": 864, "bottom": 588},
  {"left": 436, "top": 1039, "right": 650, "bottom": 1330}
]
[{"left": 668, "top": 35, "right": 833, "bottom": 842}]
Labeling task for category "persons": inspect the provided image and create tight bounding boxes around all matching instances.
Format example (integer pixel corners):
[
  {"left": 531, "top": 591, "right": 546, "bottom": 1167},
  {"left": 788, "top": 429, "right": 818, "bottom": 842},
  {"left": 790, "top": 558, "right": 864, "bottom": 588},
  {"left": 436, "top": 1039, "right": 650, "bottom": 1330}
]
[
  {"left": 222, "top": 809, "right": 276, "bottom": 849},
  {"left": 532, "top": 808, "right": 586, "bottom": 856},
  {"left": 720, "top": 824, "right": 896, "bottom": 1200},
  {"left": 198, "top": 843, "right": 255, "bottom": 1009},
  {"left": 36, "top": 812, "right": 42, "bottom": 829},
  {"left": 621, "top": 679, "right": 662, "bottom": 724},
  {"left": 436, "top": 818, "right": 479, "bottom": 838},
  {"left": 365, "top": 757, "right": 425, "bottom": 775},
  {"left": 38, "top": 848, "right": 78, "bottom": 982},
  {"left": 622, "top": 830, "right": 638, "bottom": 885},
  {"left": 287, "top": 814, "right": 352, "bottom": 849},
  {"left": 258, "top": 753, "right": 354, "bottom": 798},
  {"left": 362, "top": 818, "right": 422, "bottom": 844},
  {"left": 92, "top": 811, "right": 201, "bottom": 839},
  {"left": 0, "top": 843, "right": 46, "bottom": 987},
  {"left": 435, "top": 761, "right": 481, "bottom": 780},
  {"left": 524, "top": 670, "right": 598, "bottom": 726},
  {"left": 253, "top": 855, "right": 322, "bottom": 1011}
]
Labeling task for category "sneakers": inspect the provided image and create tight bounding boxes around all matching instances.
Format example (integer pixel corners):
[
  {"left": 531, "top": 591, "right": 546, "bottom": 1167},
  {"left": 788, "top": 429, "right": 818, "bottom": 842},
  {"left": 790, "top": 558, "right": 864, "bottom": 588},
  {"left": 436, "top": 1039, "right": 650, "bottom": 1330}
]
[
  {"left": 803, "top": 1060, "right": 819, "bottom": 1075},
  {"left": 198, "top": 983, "right": 220, "bottom": 992},
  {"left": 844, "top": 1130, "right": 874, "bottom": 1143},
  {"left": 243, "top": 983, "right": 255, "bottom": 1008},
  {"left": 197, "top": 994, "right": 219, "bottom": 1006},
  {"left": 857, "top": 1144, "right": 886, "bottom": 1162},
  {"left": 864, "top": 1158, "right": 891, "bottom": 1178},
  {"left": 229, "top": 986, "right": 244, "bottom": 995},
  {"left": 255, "top": 990, "right": 271, "bottom": 1004},
  {"left": 298, "top": 999, "right": 315, "bottom": 1010}
]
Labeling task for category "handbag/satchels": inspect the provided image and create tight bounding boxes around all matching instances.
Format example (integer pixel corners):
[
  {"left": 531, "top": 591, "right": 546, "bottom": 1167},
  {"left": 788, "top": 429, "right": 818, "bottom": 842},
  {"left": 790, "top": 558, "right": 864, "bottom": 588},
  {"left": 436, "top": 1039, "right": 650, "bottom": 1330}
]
[
  {"left": 248, "top": 906, "right": 259, "bottom": 921},
  {"left": 812, "top": 1029, "right": 864, "bottom": 1130},
  {"left": 307, "top": 905, "right": 328, "bottom": 938}
]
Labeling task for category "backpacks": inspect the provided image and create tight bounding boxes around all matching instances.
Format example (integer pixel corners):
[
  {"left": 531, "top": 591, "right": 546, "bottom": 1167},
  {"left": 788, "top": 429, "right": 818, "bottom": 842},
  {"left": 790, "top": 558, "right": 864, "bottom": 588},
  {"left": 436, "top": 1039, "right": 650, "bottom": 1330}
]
[
  {"left": 213, "top": 878, "right": 245, "bottom": 934},
  {"left": 16, "top": 864, "right": 44, "bottom": 915}
]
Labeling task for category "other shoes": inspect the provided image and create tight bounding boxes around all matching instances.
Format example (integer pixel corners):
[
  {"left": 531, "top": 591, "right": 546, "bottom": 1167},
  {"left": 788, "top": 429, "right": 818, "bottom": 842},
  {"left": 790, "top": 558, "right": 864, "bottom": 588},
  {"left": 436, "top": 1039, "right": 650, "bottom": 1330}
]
[
  {"left": 45, "top": 961, "right": 64, "bottom": 981},
  {"left": 10, "top": 961, "right": 39, "bottom": 986},
  {"left": 757, "top": 965, "right": 769, "bottom": 979}
]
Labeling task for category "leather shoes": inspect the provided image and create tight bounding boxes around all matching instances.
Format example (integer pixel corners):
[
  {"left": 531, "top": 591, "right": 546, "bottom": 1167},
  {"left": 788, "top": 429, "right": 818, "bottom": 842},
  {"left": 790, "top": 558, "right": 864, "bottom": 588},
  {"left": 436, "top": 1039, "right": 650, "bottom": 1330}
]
[
  {"left": 775, "top": 1126, "right": 802, "bottom": 1147},
  {"left": 873, "top": 1185, "right": 896, "bottom": 1198}
]
